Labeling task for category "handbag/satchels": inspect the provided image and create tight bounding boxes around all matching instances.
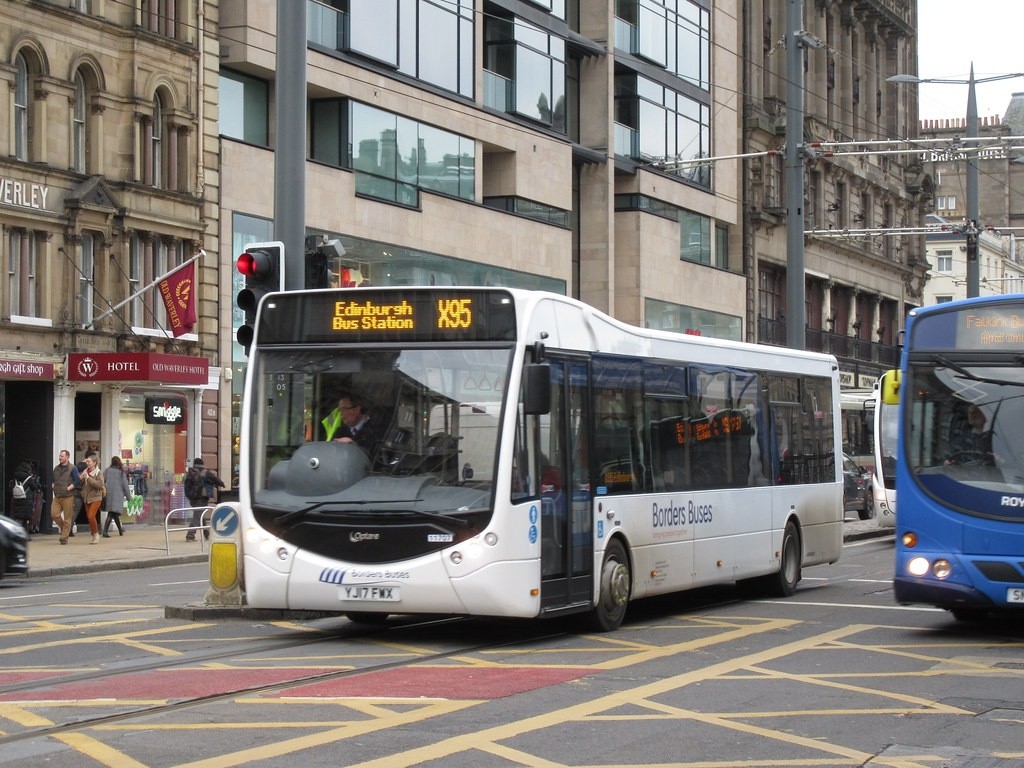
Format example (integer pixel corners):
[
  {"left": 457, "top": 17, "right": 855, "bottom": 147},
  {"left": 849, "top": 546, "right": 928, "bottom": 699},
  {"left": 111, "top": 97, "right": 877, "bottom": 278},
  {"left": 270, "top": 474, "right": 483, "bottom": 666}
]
[{"left": 100, "top": 496, "right": 106, "bottom": 512}]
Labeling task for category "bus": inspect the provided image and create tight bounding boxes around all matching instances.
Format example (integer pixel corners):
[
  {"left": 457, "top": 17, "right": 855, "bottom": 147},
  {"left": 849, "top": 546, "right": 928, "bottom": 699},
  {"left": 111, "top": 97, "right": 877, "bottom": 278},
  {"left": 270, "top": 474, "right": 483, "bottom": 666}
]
[
  {"left": 864, "top": 366, "right": 903, "bottom": 525},
  {"left": 893, "top": 289, "right": 1024, "bottom": 624},
  {"left": 212, "top": 285, "right": 848, "bottom": 637}
]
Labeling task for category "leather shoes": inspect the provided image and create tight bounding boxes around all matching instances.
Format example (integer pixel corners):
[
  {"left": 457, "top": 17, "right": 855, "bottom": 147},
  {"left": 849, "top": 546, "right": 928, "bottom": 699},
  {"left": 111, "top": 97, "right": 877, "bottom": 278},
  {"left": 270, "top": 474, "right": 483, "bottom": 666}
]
[
  {"left": 119, "top": 529, "right": 126, "bottom": 535},
  {"left": 88, "top": 533, "right": 100, "bottom": 545},
  {"left": 103, "top": 531, "right": 111, "bottom": 537}
]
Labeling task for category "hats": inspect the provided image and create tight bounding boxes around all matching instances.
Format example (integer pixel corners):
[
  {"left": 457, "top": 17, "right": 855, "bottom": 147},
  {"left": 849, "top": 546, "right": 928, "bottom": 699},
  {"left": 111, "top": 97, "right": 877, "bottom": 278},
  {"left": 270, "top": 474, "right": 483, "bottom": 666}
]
[
  {"left": 194, "top": 457, "right": 204, "bottom": 465},
  {"left": 84, "top": 446, "right": 96, "bottom": 459}
]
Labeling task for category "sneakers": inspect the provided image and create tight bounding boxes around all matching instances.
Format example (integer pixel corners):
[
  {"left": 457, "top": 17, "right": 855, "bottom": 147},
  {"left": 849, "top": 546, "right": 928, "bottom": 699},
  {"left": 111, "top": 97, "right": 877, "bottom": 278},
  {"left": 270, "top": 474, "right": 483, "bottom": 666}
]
[{"left": 186, "top": 536, "right": 198, "bottom": 541}]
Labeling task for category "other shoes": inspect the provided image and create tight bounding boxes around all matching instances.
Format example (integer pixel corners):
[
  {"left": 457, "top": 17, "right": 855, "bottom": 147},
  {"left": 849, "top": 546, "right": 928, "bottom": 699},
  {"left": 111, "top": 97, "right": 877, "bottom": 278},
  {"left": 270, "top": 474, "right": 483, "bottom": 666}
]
[{"left": 59, "top": 537, "right": 68, "bottom": 544}]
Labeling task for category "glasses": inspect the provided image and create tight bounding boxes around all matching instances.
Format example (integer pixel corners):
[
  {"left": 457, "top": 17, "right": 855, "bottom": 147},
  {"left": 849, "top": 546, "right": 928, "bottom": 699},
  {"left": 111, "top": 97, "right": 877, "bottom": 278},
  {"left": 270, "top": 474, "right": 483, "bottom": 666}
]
[{"left": 338, "top": 406, "right": 356, "bottom": 411}]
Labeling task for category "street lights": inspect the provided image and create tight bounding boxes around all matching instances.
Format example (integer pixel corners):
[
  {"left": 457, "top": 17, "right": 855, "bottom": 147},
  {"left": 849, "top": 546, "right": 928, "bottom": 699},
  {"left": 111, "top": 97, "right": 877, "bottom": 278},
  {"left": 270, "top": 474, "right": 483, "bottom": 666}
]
[{"left": 884, "top": 60, "right": 1023, "bottom": 300}]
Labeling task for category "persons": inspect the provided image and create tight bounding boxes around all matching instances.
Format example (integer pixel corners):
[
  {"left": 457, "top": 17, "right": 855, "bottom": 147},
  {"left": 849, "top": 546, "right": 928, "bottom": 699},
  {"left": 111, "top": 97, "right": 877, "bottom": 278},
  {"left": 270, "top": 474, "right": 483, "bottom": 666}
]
[
  {"left": 103, "top": 456, "right": 133, "bottom": 537},
  {"left": 944, "top": 403, "right": 1007, "bottom": 467},
  {"left": 80, "top": 454, "right": 104, "bottom": 544},
  {"left": 51, "top": 450, "right": 79, "bottom": 544},
  {"left": 334, "top": 393, "right": 376, "bottom": 453},
  {"left": 158, "top": 260, "right": 197, "bottom": 337},
  {"left": 519, "top": 449, "right": 563, "bottom": 492},
  {"left": 233, "top": 437, "right": 240, "bottom": 464},
  {"left": 68, "top": 450, "right": 96, "bottom": 537},
  {"left": 10, "top": 462, "right": 41, "bottom": 527},
  {"left": 185, "top": 458, "right": 225, "bottom": 541}
]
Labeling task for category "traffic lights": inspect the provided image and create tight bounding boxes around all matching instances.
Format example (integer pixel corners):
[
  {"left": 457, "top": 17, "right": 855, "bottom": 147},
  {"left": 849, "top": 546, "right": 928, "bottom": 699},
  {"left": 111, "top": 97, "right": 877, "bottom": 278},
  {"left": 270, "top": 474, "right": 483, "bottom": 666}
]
[{"left": 237, "top": 243, "right": 281, "bottom": 352}]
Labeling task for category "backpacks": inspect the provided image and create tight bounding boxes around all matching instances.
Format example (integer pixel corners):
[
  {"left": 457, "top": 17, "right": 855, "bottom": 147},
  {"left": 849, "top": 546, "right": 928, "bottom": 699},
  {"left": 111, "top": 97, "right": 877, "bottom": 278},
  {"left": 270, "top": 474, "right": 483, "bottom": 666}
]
[
  {"left": 12, "top": 476, "right": 33, "bottom": 498},
  {"left": 101, "top": 483, "right": 107, "bottom": 498},
  {"left": 185, "top": 468, "right": 208, "bottom": 499}
]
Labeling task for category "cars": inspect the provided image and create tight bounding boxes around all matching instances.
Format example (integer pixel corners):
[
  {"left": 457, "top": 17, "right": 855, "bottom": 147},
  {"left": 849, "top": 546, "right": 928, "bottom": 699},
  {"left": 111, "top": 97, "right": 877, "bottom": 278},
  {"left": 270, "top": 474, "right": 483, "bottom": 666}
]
[
  {"left": 0, "top": 515, "right": 29, "bottom": 580},
  {"left": 843, "top": 453, "right": 875, "bottom": 520}
]
